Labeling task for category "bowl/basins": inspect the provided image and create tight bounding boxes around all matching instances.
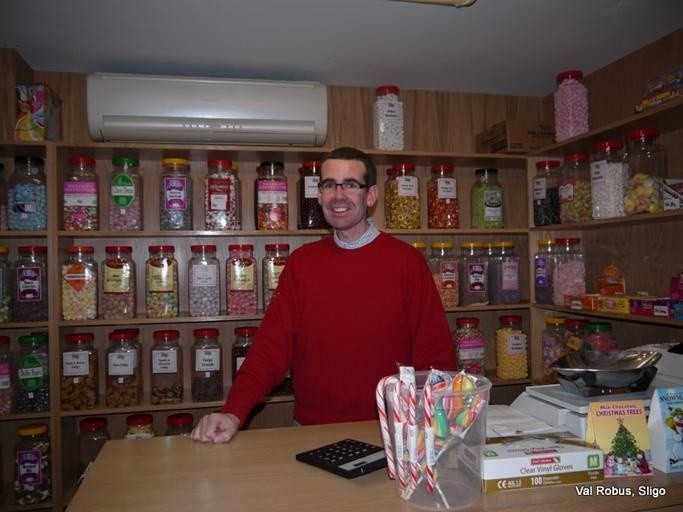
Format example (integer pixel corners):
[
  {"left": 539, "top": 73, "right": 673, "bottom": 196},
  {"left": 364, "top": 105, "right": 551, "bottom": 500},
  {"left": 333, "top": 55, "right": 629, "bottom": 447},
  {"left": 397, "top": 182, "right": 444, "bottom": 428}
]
[{"left": 546, "top": 350, "right": 659, "bottom": 393}]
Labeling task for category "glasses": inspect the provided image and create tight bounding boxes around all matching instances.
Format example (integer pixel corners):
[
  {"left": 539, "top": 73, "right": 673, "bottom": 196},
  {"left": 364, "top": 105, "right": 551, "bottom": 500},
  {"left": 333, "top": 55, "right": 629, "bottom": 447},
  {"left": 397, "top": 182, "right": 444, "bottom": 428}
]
[{"left": 317, "top": 180, "right": 367, "bottom": 192}]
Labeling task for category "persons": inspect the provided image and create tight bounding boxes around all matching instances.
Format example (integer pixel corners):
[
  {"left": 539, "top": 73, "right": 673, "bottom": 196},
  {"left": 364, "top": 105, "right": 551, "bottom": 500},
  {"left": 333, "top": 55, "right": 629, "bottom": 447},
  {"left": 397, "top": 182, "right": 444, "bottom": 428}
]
[{"left": 188, "top": 146, "right": 462, "bottom": 445}]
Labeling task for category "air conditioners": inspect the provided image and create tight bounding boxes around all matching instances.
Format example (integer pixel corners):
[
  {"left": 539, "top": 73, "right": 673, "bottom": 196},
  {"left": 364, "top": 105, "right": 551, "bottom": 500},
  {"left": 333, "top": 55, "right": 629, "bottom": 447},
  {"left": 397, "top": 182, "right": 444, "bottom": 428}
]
[{"left": 84, "top": 71, "right": 329, "bottom": 145}]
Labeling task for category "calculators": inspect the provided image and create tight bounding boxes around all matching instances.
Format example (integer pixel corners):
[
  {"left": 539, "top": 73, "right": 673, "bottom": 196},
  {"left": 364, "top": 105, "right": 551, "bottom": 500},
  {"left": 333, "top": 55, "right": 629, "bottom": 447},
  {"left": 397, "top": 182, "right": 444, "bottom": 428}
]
[{"left": 296, "top": 439, "right": 387, "bottom": 479}]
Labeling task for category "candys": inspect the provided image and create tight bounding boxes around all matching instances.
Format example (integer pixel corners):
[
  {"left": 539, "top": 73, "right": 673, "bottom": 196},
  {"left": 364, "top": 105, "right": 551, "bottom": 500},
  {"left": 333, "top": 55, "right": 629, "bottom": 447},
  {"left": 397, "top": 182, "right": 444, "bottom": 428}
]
[
  {"left": 375, "top": 162, "right": 661, "bottom": 509},
  {"left": 0, "top": 167, "right": 329, "bottom": 413},
  {"left": 13, "top": 440, "right": 51, "bottom": 505},
  {"left": 123, "top": 424, "right": 155, "bottom": 439},
  {"left": 553, "top": 78, "right": 590, "bottom": 143},
  {"left": 373, "top": 93, "right": 404, "bottom": 150}
]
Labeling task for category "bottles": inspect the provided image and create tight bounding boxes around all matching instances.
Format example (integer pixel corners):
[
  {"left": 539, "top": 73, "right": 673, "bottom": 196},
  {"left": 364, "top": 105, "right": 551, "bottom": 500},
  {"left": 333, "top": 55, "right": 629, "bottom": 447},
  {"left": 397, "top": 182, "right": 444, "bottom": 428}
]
[
  {"left": 540, "top": 316, "right": 614, "bottom": 385},
  {"left": 532, "top": 128, "right": 669, "bottom": 229},
  {"left": 371, "top": 85, "right": 404, "bottom": 151},
  {"left": 3, "top": 148, "right": 533, "bottom": 406},
  {"left": 12, "top": 411, "right": 196, "bottom": 504},
  {"left": 535, "top": 238, "right": 585, "bottom": 308},
  {"left": 553, "top": 70, "right": 590, "bottom": 143}
]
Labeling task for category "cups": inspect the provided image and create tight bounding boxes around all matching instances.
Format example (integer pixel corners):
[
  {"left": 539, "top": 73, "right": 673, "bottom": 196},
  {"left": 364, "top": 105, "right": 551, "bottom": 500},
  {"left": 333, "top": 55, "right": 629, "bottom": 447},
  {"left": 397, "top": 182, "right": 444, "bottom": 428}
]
[{"left": 385, "top": 372, "right": 488, "bottom": 511}]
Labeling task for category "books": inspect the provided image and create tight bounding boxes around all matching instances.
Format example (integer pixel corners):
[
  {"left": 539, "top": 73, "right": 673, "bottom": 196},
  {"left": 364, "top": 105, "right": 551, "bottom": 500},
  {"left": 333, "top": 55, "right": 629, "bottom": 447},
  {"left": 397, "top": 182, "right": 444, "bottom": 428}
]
[{"left": 479, "top": 400, "right": 568, "bottom": 446}]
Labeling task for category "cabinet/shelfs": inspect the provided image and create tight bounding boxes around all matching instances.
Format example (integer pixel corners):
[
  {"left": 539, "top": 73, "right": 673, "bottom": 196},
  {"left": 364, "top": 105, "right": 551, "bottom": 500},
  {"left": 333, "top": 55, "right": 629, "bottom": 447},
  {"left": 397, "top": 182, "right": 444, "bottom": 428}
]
[
  {"left": 56, "top": 141, "right": 530, "bottom": 511},
  {"left": 530, "top": 96, "right": 683, "bottom": 395},
  {"left": 0, "top": 141, "right": 55, "bottom": 512}
]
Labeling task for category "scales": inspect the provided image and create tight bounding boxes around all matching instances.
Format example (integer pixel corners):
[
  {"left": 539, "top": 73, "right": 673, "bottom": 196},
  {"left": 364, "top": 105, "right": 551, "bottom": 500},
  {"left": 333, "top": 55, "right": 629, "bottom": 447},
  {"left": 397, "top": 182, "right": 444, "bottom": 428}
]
[{"left": 510, "top": 351, "right": 661, "bottom": 440}]
